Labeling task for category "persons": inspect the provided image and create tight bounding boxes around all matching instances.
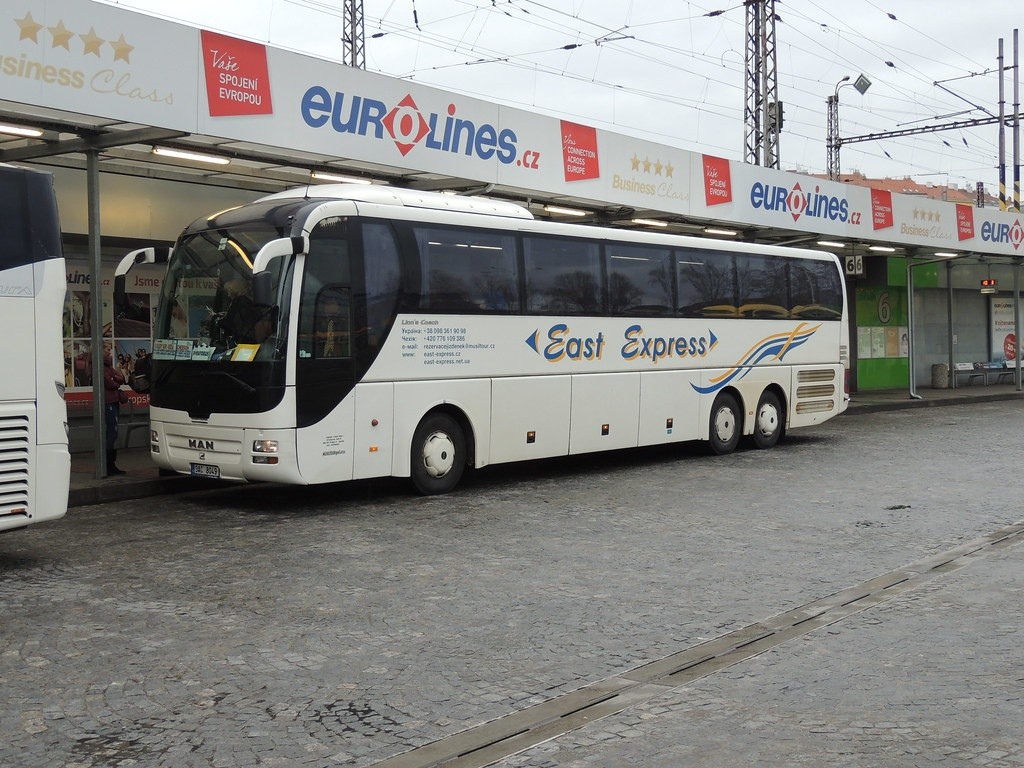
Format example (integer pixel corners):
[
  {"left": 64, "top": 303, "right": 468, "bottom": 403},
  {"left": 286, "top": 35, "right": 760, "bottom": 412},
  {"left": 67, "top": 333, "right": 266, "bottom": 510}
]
[
  {"left": 204, "top": 276, "right": 258, "bottom": 348},
  {"left": 135, "top": 348, "right": 148, "bottom": 357},
  {"left": 171, "top": 300, "right": 187, "bottom": 337},
  {"left": 125, "top": 354, "right": 135, "bottom": 374},
  {"left": 314, "top": 298, "right": 344, "bottom": 353},
  {"left": 116, "top": 354, "right": 130, "bottom": 384},
  {"left": 84, "top": 337, "right": 127, "bottom": 477}
]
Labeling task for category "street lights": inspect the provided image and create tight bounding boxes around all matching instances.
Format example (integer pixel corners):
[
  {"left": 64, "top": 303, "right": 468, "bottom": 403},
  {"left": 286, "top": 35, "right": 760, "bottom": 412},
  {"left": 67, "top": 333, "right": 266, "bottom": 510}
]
[{"left": 832, "top": 73, "right": 872, "bottom": 185}]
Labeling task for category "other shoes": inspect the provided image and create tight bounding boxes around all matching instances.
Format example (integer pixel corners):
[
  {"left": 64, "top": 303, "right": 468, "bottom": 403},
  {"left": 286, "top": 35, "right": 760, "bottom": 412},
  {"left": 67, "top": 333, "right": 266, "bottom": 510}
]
[{"left": 106, "top": 463, "right": 127, "bottom": 475}]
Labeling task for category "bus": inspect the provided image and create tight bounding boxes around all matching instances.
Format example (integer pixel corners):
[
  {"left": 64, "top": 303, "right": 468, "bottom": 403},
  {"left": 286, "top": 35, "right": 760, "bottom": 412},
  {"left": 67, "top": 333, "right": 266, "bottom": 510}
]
[
  {"left": 1, "top": 160, "right": 73, "bottom": 535},
  {"left": 114, "top": 181, "right": 852, "bottom": 496}
]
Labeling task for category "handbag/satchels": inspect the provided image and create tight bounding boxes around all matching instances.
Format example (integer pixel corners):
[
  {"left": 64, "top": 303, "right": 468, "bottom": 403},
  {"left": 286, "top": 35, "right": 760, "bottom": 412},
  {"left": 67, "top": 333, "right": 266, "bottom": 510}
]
[{"left": 119, "top": 389, "right": 129, "bottom": 404}]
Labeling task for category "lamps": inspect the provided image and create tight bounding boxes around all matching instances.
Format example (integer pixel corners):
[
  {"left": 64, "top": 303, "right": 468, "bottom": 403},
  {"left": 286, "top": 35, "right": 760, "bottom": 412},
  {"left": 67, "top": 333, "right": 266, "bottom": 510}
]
[
  {"left": 150, "top": 144, "right": 232, "bottom": 166},
  {"left": 628, "top": 218, "right": 667, "bottom": 228},
  {"left": 869, "top": 245, "right": 895, "bottom": 253},
  {"left": 441, "top": 188, "right": 510, "bottom": 201},
  {"left": 705, "top": 227, "right": 739, "bottom": 238},
  {"left": 816, "top": 240, "right": 848, "bottom": 248},
  {"left": 310, "top": 169, "right": 373, "bottom": 186},
  {"left": 934, "top": 252, "right": 959, "bottom": 258},
  {"left": 543, "top": 204, "right": 588, "bottom": 218},
  {"left": 0, "top": 123, "right": 61, "bottom": 144}
]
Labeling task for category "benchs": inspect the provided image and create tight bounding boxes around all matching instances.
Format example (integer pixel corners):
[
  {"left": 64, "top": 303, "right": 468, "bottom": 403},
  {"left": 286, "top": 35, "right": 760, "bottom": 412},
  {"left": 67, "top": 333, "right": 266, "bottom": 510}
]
[
  {"left": 1003, "top": 359, "right": 1024, "bottom": 384},
  {"left": 969, "top": 362, "right": 1015, "bottom": 385},
  {"left": 945, "top": 362, "right": 987, "bottom": 388}
]
